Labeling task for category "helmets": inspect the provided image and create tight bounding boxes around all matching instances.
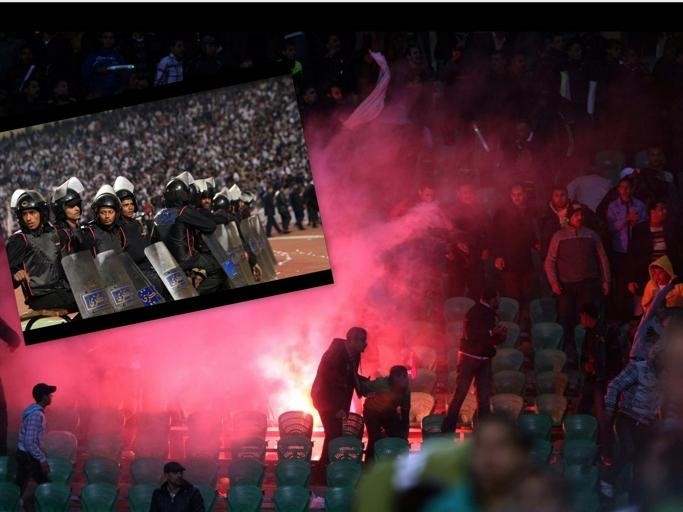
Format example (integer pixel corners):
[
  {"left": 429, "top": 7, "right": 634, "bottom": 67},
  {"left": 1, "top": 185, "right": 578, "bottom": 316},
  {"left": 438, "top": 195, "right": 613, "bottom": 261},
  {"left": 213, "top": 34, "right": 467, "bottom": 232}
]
[{"left": 9, "top": 171, "right": 257, "bottom": 234}]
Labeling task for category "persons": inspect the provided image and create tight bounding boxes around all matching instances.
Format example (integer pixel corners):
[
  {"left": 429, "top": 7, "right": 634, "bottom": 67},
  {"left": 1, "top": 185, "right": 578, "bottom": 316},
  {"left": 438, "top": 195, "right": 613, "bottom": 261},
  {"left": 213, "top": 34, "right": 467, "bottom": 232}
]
[{"left": 2, "top": 3, "right": 683, "bottom": 511}]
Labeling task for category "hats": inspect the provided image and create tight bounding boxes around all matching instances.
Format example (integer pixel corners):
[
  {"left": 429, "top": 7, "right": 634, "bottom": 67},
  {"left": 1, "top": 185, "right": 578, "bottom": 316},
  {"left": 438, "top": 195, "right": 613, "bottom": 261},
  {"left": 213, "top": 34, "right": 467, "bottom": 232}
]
[
  {"left": 32, "top": 383, "right": 56, "bottom": 398},
  {"left": 164, "top": 461, "right": 186, "bottom": 473},
  {"left": 564, "top": 199, "right": 586, "bottom": 218}
]
